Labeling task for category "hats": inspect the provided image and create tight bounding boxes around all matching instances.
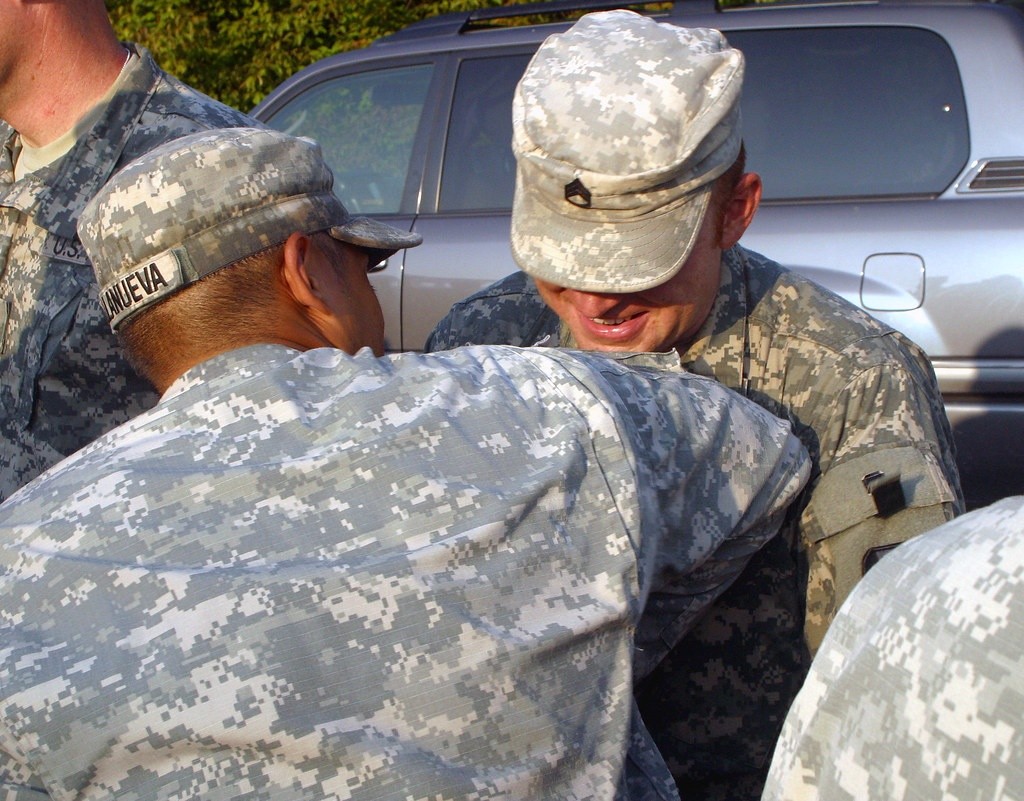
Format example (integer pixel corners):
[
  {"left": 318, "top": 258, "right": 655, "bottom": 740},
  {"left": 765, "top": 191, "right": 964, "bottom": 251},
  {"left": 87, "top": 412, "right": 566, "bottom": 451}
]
[
  {"left": 76, "top": 127, "right": 424, "bottom": 335},
  {"left": 510, "top": 8, "right": 745, "bottom": 293}
]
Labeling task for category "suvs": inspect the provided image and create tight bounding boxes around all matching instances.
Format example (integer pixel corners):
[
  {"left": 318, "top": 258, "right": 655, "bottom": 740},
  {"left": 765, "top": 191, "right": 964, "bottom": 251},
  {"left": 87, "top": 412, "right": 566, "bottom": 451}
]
[{"left": 253, "top": 0, "right": 1024, "bottom": 514}]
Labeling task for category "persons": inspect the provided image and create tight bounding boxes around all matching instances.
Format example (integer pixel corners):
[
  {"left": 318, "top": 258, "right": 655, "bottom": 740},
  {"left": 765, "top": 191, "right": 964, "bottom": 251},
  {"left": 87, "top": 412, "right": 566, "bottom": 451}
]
[
  {"left": 761, "top": 488, "right": 1024, "bottom": 801},
  {"left": 0, "top": 0, "right": 275, "bottom": 496},
  {"left": 1, "top": 127, "right": 810, "bottom": 801},
  {"left": 425, "top": 10, "right": 962, "bottom": 801}
]
